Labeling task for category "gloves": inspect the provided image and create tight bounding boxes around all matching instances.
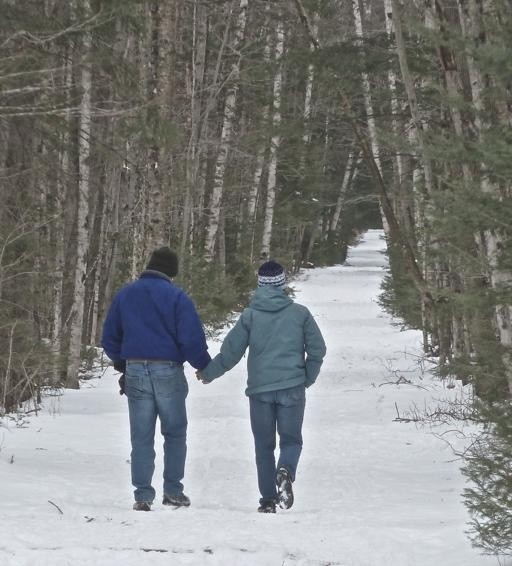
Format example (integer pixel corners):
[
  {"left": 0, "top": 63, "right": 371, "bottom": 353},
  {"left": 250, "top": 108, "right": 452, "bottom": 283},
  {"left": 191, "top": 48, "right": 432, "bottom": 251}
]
[{"left": 114, "top": 360, "right": 126, "bottom": 372}]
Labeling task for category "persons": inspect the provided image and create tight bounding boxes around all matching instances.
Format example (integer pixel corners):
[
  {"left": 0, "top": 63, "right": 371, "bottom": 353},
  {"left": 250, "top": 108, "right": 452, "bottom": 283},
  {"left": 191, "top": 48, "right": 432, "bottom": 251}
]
[
  {"left": 197, "top": 259, "right": 328, "bottom": 514},
  {"left": 102, "top": 246, "right": 210, "bottom": 511}
]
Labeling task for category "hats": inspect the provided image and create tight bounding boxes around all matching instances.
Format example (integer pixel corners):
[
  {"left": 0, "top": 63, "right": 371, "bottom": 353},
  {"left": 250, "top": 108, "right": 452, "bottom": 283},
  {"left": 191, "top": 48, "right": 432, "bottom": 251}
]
[
  {"left": 257, "top": 261, "right": 286, "bottom": 290},
  {"left": 147, "top": 247, "right": 178, "bottom": 276}
]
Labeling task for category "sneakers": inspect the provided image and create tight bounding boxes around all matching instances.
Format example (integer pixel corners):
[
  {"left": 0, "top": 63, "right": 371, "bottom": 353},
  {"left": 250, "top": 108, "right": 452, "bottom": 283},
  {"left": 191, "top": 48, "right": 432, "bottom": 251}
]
[
  {"left": 274, "top": 467, "right": 293, "bottom": 509},
  {"left": 133, "top": 502, "right": 152, "bottom": 510},
  {"left": 257, "top": 497, "right": 276, "bottom": 513},
  {"left": 162, "top": 493, "right": 190, "bottom": 506}
]
